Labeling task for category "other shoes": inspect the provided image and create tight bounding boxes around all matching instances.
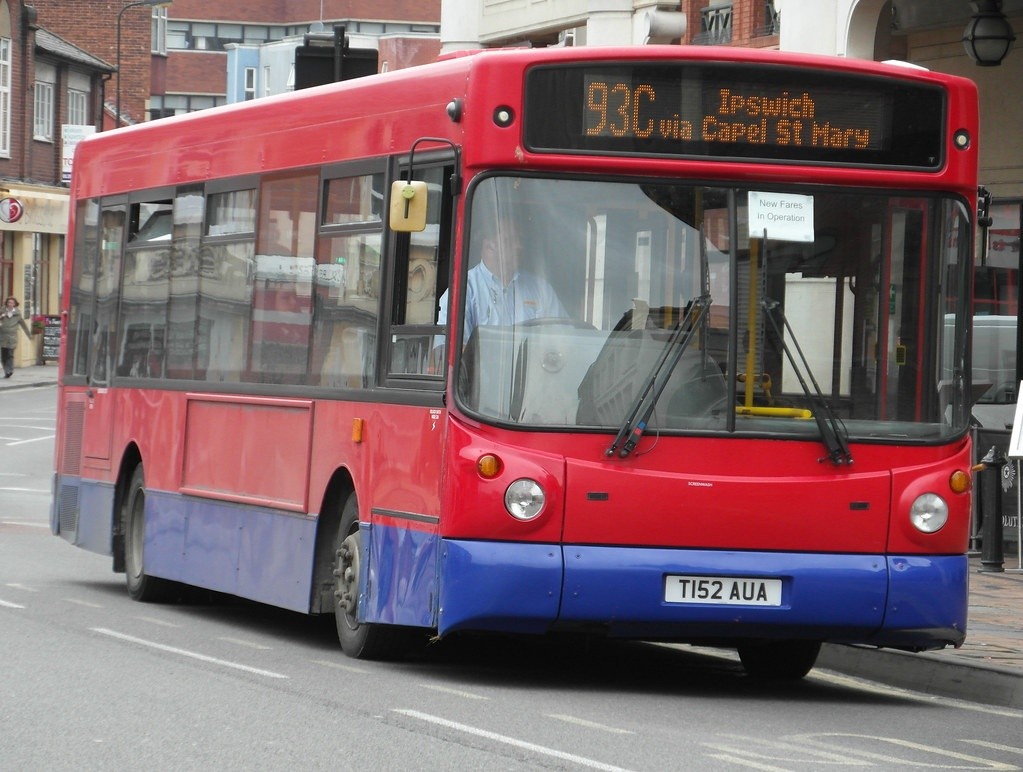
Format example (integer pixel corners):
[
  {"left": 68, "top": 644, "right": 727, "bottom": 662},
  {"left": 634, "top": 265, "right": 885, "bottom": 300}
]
[{"left": 5, "top": 372, "right": 14, "bottom": 378}]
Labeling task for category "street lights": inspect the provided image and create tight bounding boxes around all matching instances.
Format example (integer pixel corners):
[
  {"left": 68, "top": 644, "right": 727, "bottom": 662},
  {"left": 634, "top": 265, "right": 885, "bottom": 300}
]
[{"left": 115, "top": 0, "right": 175, "bottom": 127}]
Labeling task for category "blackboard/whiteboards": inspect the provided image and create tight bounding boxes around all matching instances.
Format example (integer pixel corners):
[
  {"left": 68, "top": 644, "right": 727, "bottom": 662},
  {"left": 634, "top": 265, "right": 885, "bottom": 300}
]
[{"left": 42, "top": 315, "right": 60, "bottom": 360}]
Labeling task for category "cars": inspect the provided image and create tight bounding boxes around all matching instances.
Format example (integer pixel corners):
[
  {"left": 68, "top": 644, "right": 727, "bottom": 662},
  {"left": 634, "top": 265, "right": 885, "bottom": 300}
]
[{"left": 877, "top": 314, "right": 1023, "bottom": 553}]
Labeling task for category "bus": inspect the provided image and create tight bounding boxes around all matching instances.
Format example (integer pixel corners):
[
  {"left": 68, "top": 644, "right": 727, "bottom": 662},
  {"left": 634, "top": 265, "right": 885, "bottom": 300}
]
[{"left": 55, "top": 42, "right": 993, "bottom": 687}]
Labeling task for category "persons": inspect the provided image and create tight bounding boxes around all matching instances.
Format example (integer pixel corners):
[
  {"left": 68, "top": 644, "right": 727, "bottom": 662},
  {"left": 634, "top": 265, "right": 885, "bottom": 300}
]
[
  {"left": 431, "top": 216, "right": 569, "bottom": 372},
  {"left": 261, "top": 279, "right": 310, "bottom": 373},
  {"left": 0, "top": 297, "right": 32, "bottom": 378}
]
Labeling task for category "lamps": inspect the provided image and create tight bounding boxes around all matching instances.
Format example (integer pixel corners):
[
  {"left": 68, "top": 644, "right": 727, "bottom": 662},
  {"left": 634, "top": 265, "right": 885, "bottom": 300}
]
[{"left": 964, "top": 1, "right": 1017, "bottom": 67}]
